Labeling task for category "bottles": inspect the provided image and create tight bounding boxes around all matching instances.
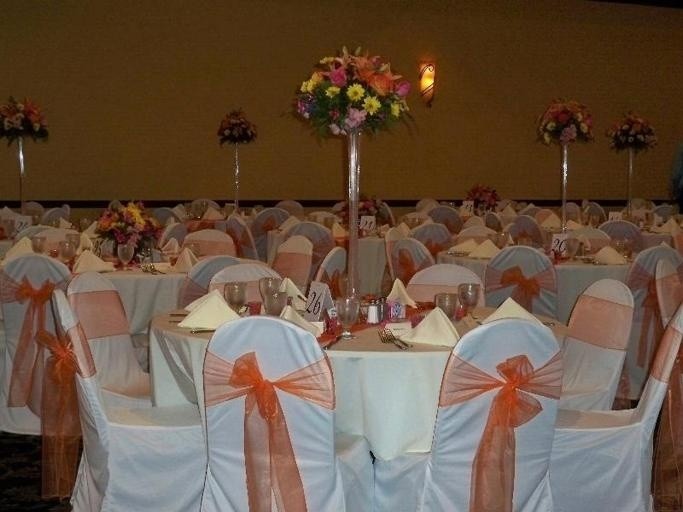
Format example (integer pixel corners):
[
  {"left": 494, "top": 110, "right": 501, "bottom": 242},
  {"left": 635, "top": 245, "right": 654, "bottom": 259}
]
[{"left": 366, "top": 297, "right": 386, "bottom": 324}]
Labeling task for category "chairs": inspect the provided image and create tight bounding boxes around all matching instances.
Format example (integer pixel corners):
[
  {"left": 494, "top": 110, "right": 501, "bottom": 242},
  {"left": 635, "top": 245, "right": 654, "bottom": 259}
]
[
  {"left": 199, "top": 316, "right": 374, "bottom": 512},
  {"left": 549, "top": 305, "right": 683, "bottom": 512},
  {"left": 49, "top": 290, "right": 201, "bottom": 512},
  {"left": 374, "top": 318, "right": 565, "bottom": 511}
]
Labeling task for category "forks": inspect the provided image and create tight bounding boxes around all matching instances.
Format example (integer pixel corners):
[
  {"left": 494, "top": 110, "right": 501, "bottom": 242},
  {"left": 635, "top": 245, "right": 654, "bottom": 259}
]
[{"left": 378, "top": 327, "right": 409, "bottom": 350}]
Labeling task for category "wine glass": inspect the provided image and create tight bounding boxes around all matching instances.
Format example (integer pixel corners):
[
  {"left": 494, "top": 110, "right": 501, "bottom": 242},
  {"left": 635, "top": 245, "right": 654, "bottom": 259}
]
[
  {"left": 436, "top": 293, "right": 458, "bottom": 323},
  {"left": 223, "top": 277, "right": 288, "bottom": 318},
  {"left": 335, "top": 271, "right": 361, "bottom": 340},
  {"left": 485, "top": 230, "right": 634, "bottom": 263},
  {"left": 458, "top": 283, "right": 481, "bottom": 319},
  {"left": 2, "top": 219, "right": 135, "bottom": 272}
]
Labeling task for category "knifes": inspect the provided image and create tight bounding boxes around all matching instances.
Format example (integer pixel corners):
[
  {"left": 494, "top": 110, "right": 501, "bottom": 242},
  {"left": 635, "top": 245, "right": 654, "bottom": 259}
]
[{"left": 323, "top": 335, "right": 341, "bottom": 350}]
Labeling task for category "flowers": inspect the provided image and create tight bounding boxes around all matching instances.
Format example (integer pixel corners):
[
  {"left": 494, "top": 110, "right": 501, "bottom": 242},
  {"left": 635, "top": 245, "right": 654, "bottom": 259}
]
[
  {"left": 295, "top": 46, "right": 417, "bottom": 138},
  {"left": 0, "top": 95, "right": 49, "bottom": 145},
  {"left": 216, "top": 109, "right": 256, "bottom": 145},
  {"left": 606, "top": 109, "right": 656, "bottom": 153},
  {"left": 468, "top": 184, "right": 498, "bottom": 211},
  {"left": 537, "top": 99, "right": 594, "bottom": 146}
]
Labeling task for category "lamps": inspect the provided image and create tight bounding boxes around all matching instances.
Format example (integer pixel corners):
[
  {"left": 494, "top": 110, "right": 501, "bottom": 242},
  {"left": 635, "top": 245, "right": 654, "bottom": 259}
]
[{"left": 419, "top": 61, "right": 434, "bottom": 106}]
